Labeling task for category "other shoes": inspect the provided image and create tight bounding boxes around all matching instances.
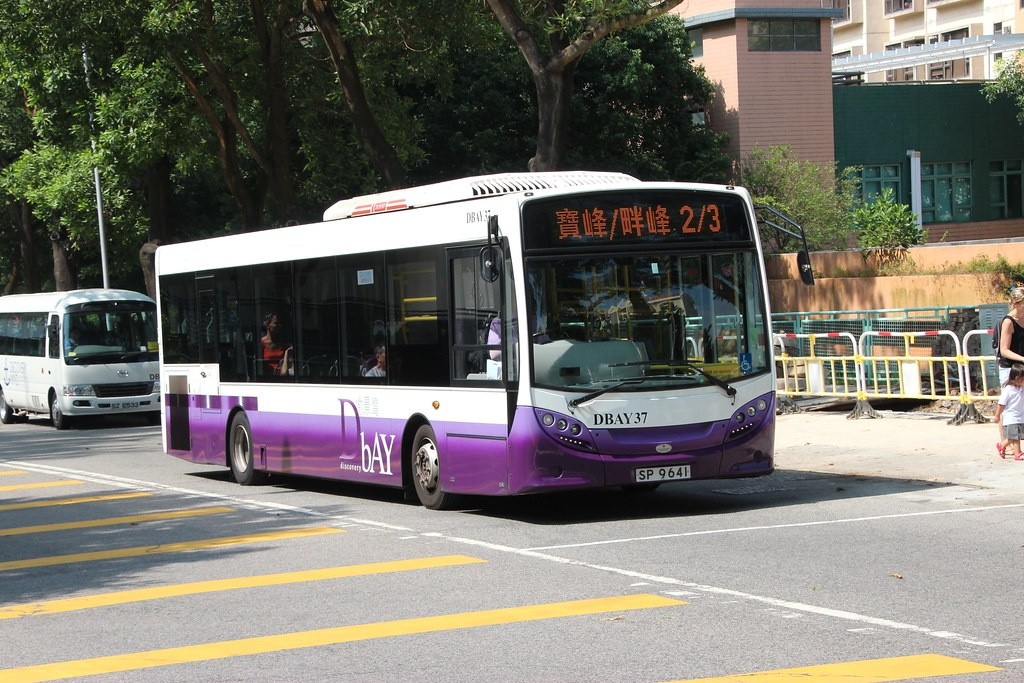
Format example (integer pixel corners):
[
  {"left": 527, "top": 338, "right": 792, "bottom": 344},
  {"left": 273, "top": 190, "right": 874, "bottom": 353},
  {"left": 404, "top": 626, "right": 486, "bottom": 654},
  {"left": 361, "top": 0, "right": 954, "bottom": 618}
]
[{"left": 1004, "top": 444, "right": 1014, "bottom": 455}]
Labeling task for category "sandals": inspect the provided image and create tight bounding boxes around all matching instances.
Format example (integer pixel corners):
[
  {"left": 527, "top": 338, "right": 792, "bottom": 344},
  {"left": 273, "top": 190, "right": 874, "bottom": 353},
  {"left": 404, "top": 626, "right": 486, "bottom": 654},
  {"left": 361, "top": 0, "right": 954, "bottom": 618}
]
[
  {"left": 1014, "top": 452, "right": 1024, "bottom": 460},
  {"left": 996, "top": 442, "right": 1006, "bottom": 459}
]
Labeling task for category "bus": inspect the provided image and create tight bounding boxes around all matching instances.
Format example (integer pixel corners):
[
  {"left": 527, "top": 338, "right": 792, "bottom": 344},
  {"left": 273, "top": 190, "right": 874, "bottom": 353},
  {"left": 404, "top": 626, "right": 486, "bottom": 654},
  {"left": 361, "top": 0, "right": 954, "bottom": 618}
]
[
  {"left": 0, "top": 288, "right": 162, "bottom": 431},
  {"left": 153, "top": 170, "right": 817, "bottom": 512}
]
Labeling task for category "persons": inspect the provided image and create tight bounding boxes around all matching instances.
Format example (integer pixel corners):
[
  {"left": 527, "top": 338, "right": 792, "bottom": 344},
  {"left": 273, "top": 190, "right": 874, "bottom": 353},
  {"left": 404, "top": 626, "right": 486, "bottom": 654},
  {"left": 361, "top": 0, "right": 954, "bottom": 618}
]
[
  {"left": 260, "top": 313, "right": 295, "bottom": 376},
  {"left": 994, "top": 364, "right": 1024, "bottom": 460},
  {"left": 365, "top": 344, "right": 386, "bottom": 378},
  {"left": 486, "top": 291, "right": 550, "bottom": 361},
  {"left": 998, "top": 288, "right": 1024, "bottom": 455},
  {"left": 67, "top": 326, "right": 87, "bottom": 348}
]
